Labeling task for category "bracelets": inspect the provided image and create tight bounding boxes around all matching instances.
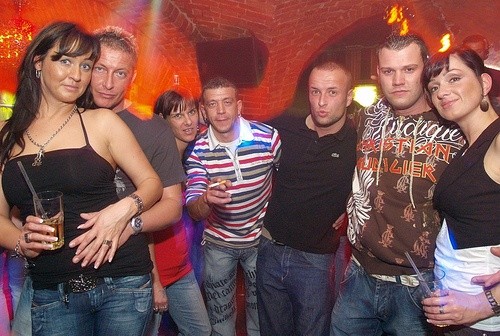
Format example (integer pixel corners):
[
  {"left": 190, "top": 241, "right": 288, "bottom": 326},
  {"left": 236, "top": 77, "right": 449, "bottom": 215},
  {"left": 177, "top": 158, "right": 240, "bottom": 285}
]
[
  {"left": 486, "top": 289, "right": 500, "bottom": 314},
  {"left": 15, "top": 239, "right": 24, "bottom": 257},
  {"left": 127, "top": 195, "right": 144, "bottom": 217}
]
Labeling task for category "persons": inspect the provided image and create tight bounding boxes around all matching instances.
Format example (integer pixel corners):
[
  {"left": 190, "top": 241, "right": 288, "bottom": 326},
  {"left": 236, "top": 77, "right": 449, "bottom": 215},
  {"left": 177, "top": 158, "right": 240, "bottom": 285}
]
[
  {"left": 14, "top": 26, "right": 187, "bottom": 336},
  {"left": 421, "top": 46, "right": 500, "bottom": 336},
  {"left": 150, "top": 86, "right": 211, "bottom": 336},
  {"left": 0, "top": 21, "right": 163, "bottom": 336},
  {"left": 184, "top": 77, "right": 282, "bottom": 336},
  {"left": 256, "top": 61, "right": 357, "bottom": 336},
  {"left": 329, "top": 33, "right": 500, "bottom": 336}
]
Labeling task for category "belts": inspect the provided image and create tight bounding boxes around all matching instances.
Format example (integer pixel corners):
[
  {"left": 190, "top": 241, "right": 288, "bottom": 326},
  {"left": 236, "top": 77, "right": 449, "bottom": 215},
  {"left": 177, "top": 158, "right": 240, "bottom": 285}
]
[
  {"left": 352, "top": 252, "right": 425, "bottom": 288},
  {"left": 260, "top": 225, "right": 286, "bottom": 246},
  {"left": 32, "top": 274, "right": 120, "bottom": 293}
]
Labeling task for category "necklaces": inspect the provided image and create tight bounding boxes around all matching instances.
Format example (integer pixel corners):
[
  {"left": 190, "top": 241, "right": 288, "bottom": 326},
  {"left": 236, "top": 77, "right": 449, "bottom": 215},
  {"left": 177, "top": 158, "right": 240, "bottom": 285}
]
[{"left": 25, "top": 107, "right": 77, "bottom": 166}]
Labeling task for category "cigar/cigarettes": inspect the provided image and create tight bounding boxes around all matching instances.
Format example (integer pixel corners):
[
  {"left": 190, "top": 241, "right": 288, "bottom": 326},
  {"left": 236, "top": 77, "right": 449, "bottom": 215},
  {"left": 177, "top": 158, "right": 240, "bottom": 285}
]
[{"left": 209, "top": 180, "right": 227, "bottom": 187}]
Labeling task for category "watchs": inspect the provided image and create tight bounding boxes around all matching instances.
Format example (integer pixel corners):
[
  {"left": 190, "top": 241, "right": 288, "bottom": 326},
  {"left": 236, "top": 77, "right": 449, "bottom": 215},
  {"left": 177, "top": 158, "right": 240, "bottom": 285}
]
[{"left": 132, "top": 216, "right": 144, "bottom": 236}]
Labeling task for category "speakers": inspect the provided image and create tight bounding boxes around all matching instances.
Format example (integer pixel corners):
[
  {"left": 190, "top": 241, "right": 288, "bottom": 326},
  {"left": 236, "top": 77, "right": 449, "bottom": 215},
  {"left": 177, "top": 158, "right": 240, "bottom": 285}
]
[{"left": 196, "top": 37, "right": 268, "bottom": 88}]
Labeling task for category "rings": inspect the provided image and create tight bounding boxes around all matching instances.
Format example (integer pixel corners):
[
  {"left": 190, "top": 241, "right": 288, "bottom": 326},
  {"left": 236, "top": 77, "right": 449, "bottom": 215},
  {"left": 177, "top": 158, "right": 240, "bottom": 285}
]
[
  {"left": 159, "top": 307, "right": 163, "bottom": 310},
  {"left": 25, "top": 232, "right": 32, "bottom": 243},
  {"left": 440, "top": 305, "right": 444, "bottom": 314},
  {"left": 153, "top": 307, "right": 158, "bottom": 310},
  {"left": 164, "top": 306, "right": 167, "bottom": 309},
  {"left": 103, "top": 240, "right": 111, "bottom": 246}
]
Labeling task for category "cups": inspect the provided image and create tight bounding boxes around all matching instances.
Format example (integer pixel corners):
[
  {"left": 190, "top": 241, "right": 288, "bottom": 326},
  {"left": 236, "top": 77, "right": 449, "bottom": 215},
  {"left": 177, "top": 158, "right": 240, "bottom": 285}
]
[
  {"left": 418, "top": 269, "right": 451, "bottom": 327},
  {"left": 32, "top": 193, "right": 64, "bottom": 251}
]
